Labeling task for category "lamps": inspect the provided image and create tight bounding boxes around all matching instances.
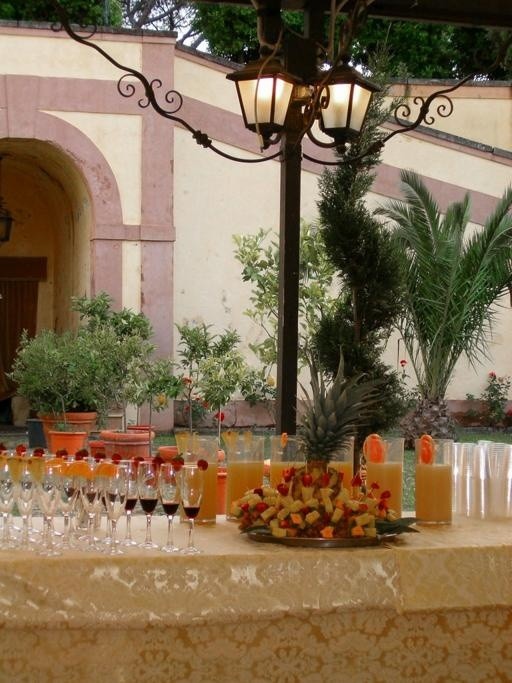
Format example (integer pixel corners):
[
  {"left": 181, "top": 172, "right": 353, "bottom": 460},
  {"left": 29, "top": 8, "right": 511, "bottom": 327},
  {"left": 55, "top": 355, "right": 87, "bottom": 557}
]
[
  {"left": 0, "top": 198, "right": 15, "bottom": 242},
  {"left": 226, "top": 53, "right": 384, "bottom": 155}
]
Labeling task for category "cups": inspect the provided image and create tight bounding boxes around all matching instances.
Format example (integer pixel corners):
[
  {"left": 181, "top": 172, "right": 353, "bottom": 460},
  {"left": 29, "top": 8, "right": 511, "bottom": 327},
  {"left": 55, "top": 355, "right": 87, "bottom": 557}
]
[
  {"left": 364, "top": 437, "right": 406, "bottom": 516},
  {"left": 181, "top": 435, "right": 220, "bottom": 528},
  {"left": 413, "top": 437, "right": 453, "bottom": 527},
  {"left": 325, "top": 436, "right": 356, "bottom": 491},
  {"left": 226, "top": 435, "right": 265, "bottom": 522},
  {"left": 270, "top": 435, "right": 306, "bottom": 490},
  {"left": 445, "top": 436, "right": 511, "bottom": 518}
]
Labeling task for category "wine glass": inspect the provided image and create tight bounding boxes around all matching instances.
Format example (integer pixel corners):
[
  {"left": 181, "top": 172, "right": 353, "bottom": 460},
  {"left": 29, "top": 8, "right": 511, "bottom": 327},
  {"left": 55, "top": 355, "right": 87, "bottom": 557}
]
[{"left": 1, "top": 462, "right": 204, "bottom": 554}]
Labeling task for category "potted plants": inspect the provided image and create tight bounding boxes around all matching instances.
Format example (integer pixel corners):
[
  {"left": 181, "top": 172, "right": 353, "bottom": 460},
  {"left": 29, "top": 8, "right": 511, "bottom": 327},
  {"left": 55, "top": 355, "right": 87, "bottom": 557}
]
[
  {"left": 372, "top": 168, "right": 512, "bottom": 450},
  {"left": 4, "top": 291, "right": 224, "bottom": 461}
]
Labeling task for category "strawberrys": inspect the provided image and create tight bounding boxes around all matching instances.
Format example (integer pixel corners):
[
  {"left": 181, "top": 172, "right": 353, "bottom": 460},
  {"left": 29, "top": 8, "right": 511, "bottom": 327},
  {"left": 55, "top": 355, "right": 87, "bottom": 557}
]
[{"left": 15, "top": 443, "right": 208, "bottom": 471}]
